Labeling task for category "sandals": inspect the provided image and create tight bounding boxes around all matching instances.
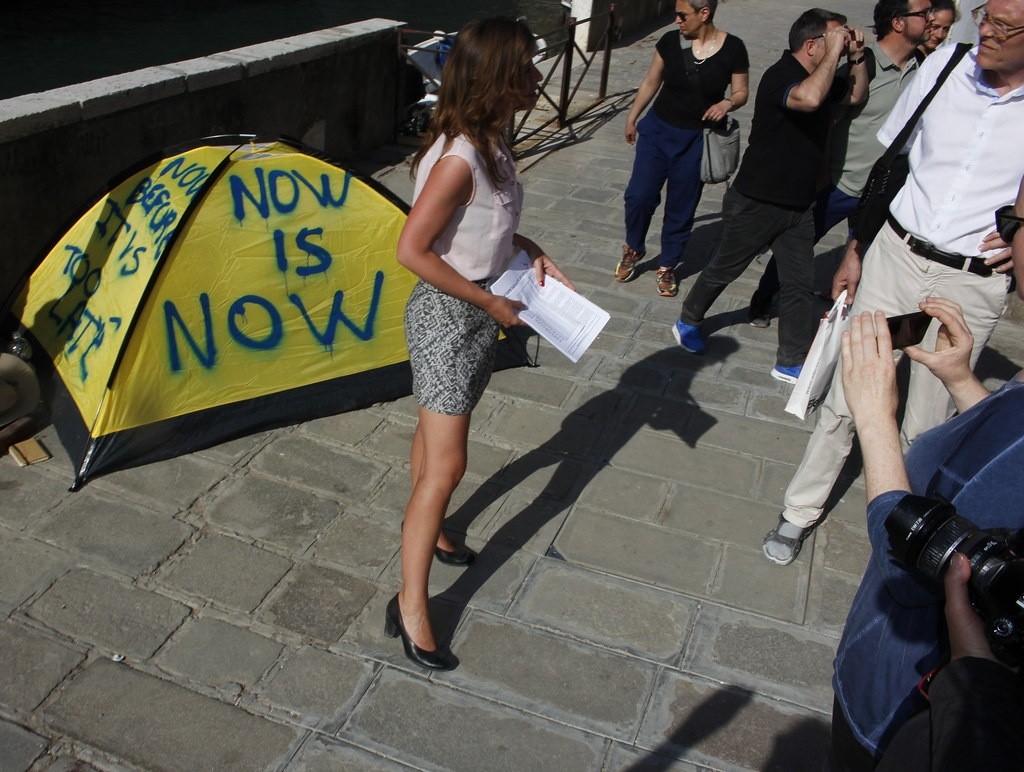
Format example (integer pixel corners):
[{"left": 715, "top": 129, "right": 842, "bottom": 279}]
[{"left": 761, "top": 511, "right": 813, "bottom": 566}]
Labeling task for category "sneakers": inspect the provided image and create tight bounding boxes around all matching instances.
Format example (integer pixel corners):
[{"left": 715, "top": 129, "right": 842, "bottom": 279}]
[
  {"left": 748, "top": 302, "right": 770, "bottom": 327},
  {"left": 654, "top": 267, "right": 678, "bottom": 296},
  {"left": 615, "top": 244, "right": 646, "bottom": 283},
  {"left": 673, "top": 318, "right": 703, "bottom": 352},
  {"left": 770, "top": 362, "right": 802, "bottom": 386}
]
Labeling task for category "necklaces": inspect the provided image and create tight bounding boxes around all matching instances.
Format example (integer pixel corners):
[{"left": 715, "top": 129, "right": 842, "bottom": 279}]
[{"left": 693, "top": 29, "right": 717, "bottom": 64}]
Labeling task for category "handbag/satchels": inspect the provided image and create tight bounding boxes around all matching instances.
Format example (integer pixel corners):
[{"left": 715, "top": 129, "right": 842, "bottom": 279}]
[
  {"left": 701, "top": 116, "right": 739, "bottom": 184},
  {"left": 857, "top": 154, "right": 909, "bottom": 208},
  {"left": 784, "top": 290, "right": 849, "bottom": 421}
]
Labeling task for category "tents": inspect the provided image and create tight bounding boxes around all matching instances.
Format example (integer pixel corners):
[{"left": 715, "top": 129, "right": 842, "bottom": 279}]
[{"left": 0, "top": 132, "right": 542, "bottom": 491}]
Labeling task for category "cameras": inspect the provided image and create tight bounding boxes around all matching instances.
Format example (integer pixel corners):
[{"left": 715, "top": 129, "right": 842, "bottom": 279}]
[{"left": 884, "top": 493, "right": 1024, "bottom": 669}]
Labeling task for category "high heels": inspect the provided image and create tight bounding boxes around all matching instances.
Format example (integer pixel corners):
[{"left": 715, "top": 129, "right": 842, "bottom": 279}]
[
  {"left": 401, "top": 521, "right": 475, "bottom": 567},
  {"left": 384, "top": 592, "right": 444, "bottom": 672}
]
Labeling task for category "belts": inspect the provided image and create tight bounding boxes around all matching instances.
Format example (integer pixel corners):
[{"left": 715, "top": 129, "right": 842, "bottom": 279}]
[{"left": 886, "top": 210, "right": 1011, "bottom": 277}]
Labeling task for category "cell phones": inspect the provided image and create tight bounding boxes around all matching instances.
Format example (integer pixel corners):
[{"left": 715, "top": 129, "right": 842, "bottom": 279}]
[{"left": 876, "top": 311, "right": 933, "bottom": 353}]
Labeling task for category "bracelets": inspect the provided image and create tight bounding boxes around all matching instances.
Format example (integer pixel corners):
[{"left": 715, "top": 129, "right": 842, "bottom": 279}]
[
  {"left": 848, "top": 54, "right": 865, "bottom": 65},
  {"left": 726, "top": 98, "right": 735, "bottom": 111}
]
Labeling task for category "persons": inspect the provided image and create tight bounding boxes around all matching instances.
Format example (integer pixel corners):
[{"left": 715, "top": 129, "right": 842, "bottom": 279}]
[
  {"left": 763, "top": 0, "right": 1024, "bottom": 565},
  {"left": 876, "top": 552, "right": 1024, "bottom": 772},
  {"left": 614, "top": 0, "right": 750, "bottom": 296},
  {"left": 672, "top": 8, "right": 869, "bottom": 383},
  {"left": 385, "top": 21, "right": 575, "bottom": 671},
  {"left": 831, "top": 296, "right": 1024, "bottom": 772},
  {"left": 748, "top": 0, "right": 957, "bottom": 328}
]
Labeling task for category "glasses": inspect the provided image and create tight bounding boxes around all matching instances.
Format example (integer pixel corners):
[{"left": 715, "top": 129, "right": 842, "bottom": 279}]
[
  {"left": 995, "top": 206, "right": 1024, "bottom": 244},
  {"left": 675, "top": 11, "right": 697, "bottom": 21},
  {"left": 971, "top": 3, "right": 1024, "bottom": 38},
  {"left": 899, "top": 8, "right": 931, "bottom": 22}
]
